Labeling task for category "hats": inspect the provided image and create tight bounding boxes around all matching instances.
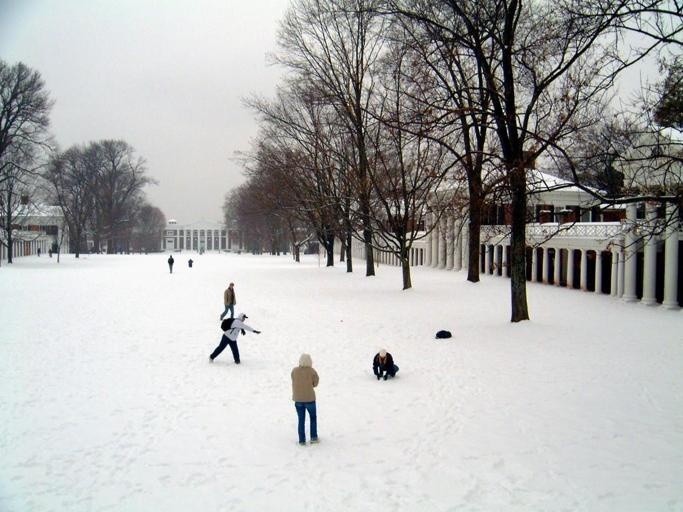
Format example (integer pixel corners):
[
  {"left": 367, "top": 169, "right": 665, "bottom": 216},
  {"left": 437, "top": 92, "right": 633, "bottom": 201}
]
[{"left": 380, "top": 349, "right": 386, "bottom": 358}]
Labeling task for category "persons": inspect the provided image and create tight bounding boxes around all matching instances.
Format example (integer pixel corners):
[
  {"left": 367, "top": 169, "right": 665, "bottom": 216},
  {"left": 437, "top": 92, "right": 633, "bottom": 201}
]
[
  {"left": 220, "top": 282, "right": 236, "bottom": 321},
  {"left": 372, "top": 348, "right": 399, "bottom": 380},
  {"left": 188, "top": 259, "right": 193, "bottom": 268},
  {"left": 37, "top": 247, "right": 41, "bottom": 257},
  {"left": 290, "top": 353, "right": 320, "bottom": 446},
  {"left": 168, "top": 255, "right": 174, "bottom": 274},
  {"left": 208, "top": 313, "right": 262, "bottom": 365},
  {"left": 48, "top": 249, "right": 52, "bottom": 257}
]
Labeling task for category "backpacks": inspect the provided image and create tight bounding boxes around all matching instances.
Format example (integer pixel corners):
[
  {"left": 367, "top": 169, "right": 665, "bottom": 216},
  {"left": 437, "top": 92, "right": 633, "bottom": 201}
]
[{"left": 221, "top": 318, "right": 235, "bottom": 331}]
